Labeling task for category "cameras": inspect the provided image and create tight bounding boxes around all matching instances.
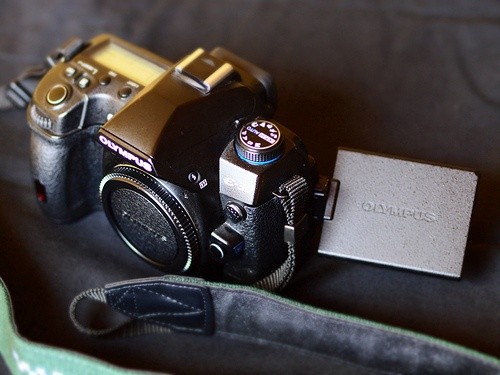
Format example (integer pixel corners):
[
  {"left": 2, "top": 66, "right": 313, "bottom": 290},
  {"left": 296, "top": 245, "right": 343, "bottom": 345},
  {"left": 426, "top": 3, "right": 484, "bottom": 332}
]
[{"left": 26, "top": 33, "right": 481, "bottom": 284}]
